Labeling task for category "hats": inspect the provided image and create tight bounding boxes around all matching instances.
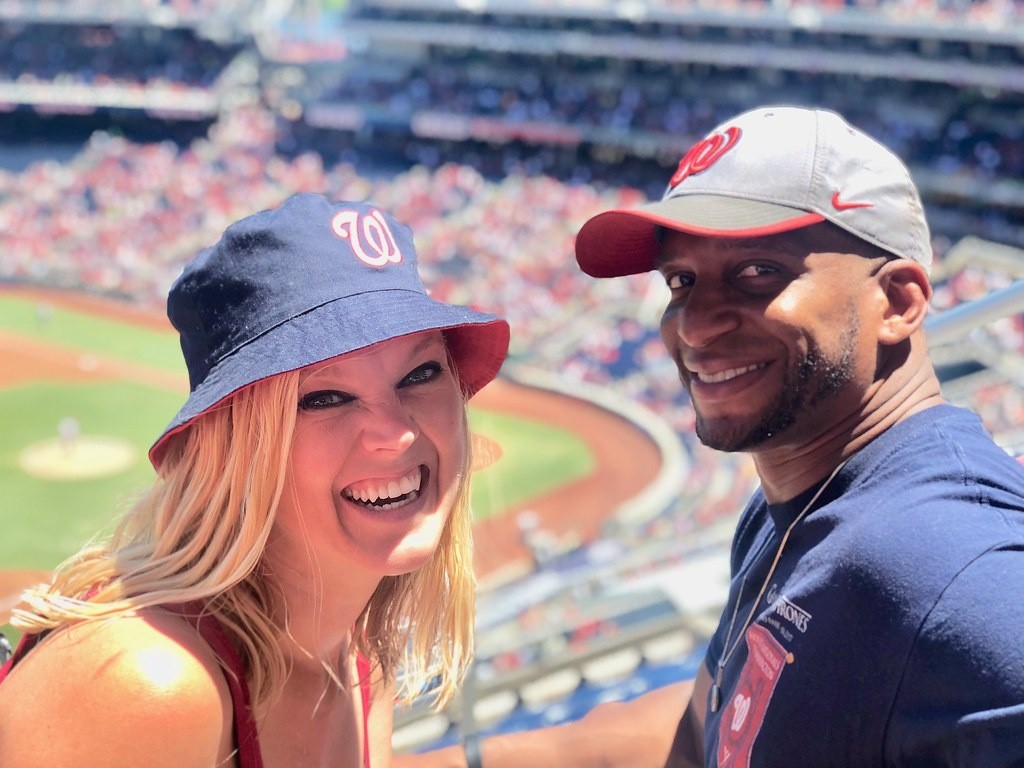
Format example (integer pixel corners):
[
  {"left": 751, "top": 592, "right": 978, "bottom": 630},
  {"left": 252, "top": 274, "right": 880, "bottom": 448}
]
[
  {"left": 575, "top": 105, "right": 934, "bottom": 279},
  {"left": 148, "top": 193, "right": 510, "bottom": 480}
]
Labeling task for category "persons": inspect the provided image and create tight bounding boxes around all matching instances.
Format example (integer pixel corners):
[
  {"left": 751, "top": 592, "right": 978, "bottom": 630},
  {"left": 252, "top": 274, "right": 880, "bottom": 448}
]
[
  {"left": 0, "top": 193, "right": 512, "bottom": 768},
  {"left": 573, "top": 105, "right": 1024, "bottom": 768}
]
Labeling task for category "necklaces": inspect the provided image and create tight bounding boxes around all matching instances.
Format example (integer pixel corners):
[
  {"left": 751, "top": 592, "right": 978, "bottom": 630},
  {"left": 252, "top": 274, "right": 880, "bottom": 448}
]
[{"left": 711, "top": 451, "right": 853, "bottom": 713}]
